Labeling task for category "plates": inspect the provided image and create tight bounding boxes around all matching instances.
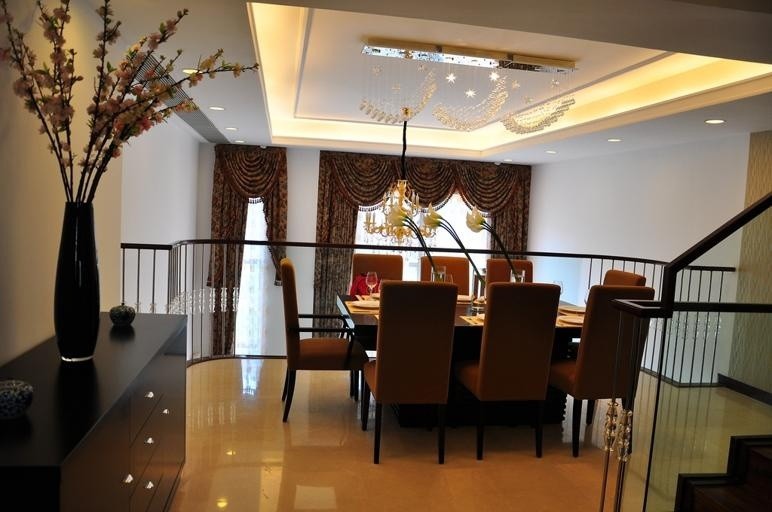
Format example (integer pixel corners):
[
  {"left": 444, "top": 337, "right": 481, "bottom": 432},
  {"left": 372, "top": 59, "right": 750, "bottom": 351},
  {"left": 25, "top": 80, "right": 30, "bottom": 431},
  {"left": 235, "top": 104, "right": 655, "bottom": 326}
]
[
  {"left": 477, "top": 314, "right": 486, "bottom": 321},
  {"left": 455, "top": 294, "right": 470, "bottom": 303},
  {"left": 351, "top": 300, "right": 380, "bottom": 309},
  {"left": 559, "top": 304, "right": 587, "bottom": 314},
  {"left": 370, "top": 292, "right": 381, "bottom": 299},
  {"left": 559, "top": 316, "right": 583, "bottom": 324}
]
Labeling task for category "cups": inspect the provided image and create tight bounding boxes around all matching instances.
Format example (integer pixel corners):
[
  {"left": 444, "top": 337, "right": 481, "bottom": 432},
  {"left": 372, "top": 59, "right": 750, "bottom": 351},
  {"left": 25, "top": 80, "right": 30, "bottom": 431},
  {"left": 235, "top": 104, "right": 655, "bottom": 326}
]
[
  {"left": 583, "top": 289, "right": 591, "bottom": 304},
  {"left": 553, "top": 280, "right": 564, "bottom": 294},
  {"left": 509, "top": 268, "right": 526, "bottom": 284},
  {"left": 471, "top": 267, "right": 487, "bottom": 310},
  {"left": 430, "top": 265, "right": 447, "bottom": 284},
  {"left": 447, "top": 273, "right": 454, "bottom": 283}
]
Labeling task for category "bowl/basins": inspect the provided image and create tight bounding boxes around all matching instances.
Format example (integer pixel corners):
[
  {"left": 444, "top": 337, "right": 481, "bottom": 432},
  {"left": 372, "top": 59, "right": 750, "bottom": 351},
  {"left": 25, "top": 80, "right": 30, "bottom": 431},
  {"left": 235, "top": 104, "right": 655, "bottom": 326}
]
[{"left": 0, "top": 380, "right": 36, "bottom": 420}]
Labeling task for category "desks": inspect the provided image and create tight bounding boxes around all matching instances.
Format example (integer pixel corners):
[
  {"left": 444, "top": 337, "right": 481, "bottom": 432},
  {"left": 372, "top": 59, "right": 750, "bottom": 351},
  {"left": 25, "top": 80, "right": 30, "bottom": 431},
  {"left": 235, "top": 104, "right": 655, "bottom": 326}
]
[{"left": 336, "top": 294, "right": 597, "bottom": 431}]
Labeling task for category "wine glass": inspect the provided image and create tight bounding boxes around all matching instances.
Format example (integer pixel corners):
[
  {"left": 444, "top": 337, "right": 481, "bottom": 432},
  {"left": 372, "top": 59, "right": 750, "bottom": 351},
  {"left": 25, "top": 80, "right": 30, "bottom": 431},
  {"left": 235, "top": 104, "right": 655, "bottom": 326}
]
[{"left": 366, "top": 271, "right": 378, "bottom": 301}]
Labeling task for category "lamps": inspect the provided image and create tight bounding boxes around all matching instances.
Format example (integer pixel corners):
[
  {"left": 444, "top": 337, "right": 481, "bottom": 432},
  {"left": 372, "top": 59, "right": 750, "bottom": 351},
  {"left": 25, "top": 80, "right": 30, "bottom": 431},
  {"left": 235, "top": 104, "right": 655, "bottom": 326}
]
[
  {"left": 359, "top": 38, "right": 576, "bottom": 135},
  {"left": 362, "top": 108, "right": 439, "bottom": 239}
]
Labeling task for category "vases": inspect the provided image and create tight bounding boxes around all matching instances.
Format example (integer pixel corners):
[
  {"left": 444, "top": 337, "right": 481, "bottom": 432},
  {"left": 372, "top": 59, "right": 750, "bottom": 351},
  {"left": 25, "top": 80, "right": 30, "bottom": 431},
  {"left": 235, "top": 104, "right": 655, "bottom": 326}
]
[{"left": 50, "top": 198, "right": 101, "bottom": 366}]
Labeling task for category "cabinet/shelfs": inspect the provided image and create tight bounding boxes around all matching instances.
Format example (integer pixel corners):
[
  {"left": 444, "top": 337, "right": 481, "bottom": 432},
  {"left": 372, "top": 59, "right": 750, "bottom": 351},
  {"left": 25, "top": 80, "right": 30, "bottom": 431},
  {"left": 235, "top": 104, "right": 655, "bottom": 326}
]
[{"left": 1, "top": 309, "right": 193, "bottom": 512}]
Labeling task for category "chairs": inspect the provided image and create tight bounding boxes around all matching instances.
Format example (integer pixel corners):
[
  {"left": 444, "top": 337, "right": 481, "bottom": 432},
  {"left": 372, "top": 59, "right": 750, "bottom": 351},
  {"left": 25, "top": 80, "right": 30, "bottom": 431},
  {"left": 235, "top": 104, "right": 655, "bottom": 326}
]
[
  {"left": 421, "top": 256, "right": 469, "bottom": 296},
  {"left": 548, "top": 284, "right": 656, "bottom": 459},
  {"left": 453, "top": 281, "right": 561, "bottom": 461},
  {"left": 486, "top": 258, "right": 533, "bottom": 293},
  {"left": 362, "top": 278, "right": 459, "bottom": 466},
  {"left": 280, "top": 258, "right": 370, "bottom": 423},
  {"left": 603, "top": 269, "right": 646, "bottom": 286},
  {"left": 348, "top": 253, "right": 403, "bottom": 296}
]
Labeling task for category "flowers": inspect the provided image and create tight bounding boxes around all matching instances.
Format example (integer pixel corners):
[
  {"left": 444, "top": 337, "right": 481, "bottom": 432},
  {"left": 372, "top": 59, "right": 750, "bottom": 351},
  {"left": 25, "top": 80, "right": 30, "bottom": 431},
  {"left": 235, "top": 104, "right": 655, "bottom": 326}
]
[
  {"left": 382, "top": 203, "right": 436, "bottom": 266},
  {"left": 418, "top": 203, "right": 483, "bottom": 275},
  {"left": 0, "top": 0, "right": 266, "bottom": 201},
  {"left": 461, "top": 205, "right": 518, "bottom": 272}
]
